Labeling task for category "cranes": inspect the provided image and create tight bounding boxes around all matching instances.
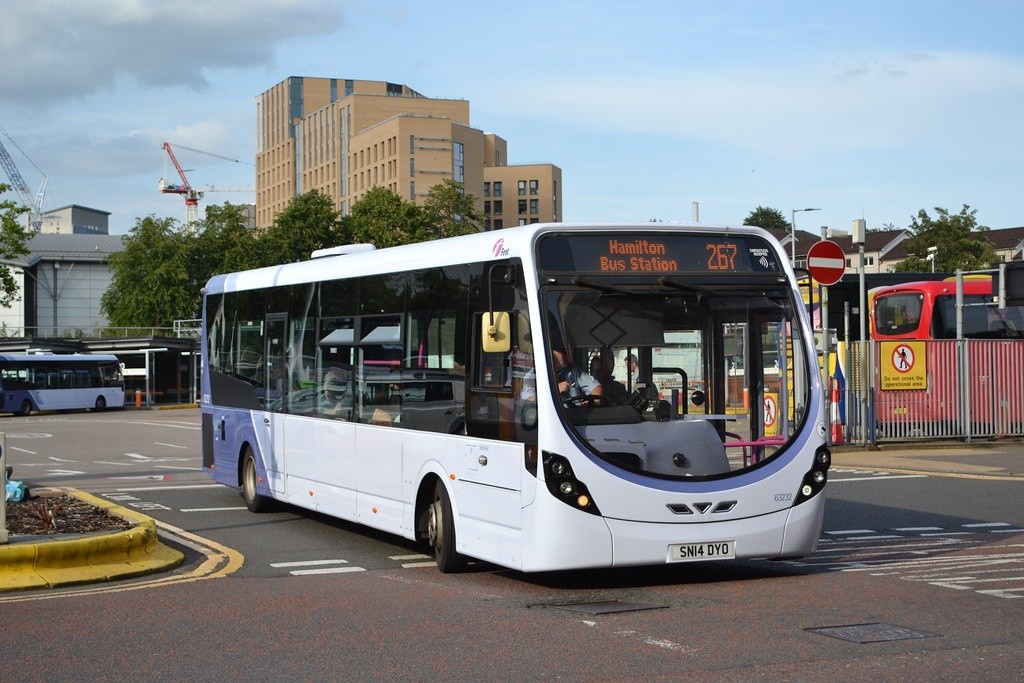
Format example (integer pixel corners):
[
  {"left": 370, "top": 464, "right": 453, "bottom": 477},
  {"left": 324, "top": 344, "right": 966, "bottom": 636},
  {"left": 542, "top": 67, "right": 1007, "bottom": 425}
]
[
  {"left": 0, "top": 128, "right": 48, "bottom": 230},
  {"left": 159, "top": 141, "right": 255, "bottom": 234}
]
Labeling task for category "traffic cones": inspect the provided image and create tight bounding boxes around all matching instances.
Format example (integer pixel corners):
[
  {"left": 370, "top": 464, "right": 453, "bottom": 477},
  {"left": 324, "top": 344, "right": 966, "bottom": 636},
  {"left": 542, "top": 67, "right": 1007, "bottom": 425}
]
[{"left": 831, "top": 391, "right": 847, "bottom": 445}]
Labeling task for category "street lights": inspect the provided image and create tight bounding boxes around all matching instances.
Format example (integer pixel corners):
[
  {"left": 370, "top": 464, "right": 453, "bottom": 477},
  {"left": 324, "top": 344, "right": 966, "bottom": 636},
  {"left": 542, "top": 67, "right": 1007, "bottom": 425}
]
[{"left": 791, "top": 207, "right": 822, "bottom": 270}]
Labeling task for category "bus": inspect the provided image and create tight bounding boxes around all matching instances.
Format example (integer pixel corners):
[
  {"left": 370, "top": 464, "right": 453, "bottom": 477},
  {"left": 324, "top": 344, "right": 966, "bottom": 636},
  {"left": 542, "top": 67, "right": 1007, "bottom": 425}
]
[
  {"left": 0, "top": 355, "right": 127, "bottom": 416},
  {"left": 211, "top": 349, "right": 315, "bottom": 406},
  {"left": 797, "top": 272, "right": 964, "bottom": 397},
  {"left": 870, "top": 273, "right": 1024, "bottom": 436},
  {"left": 201, "top": 220, "right": 831, "bottom": 572}
]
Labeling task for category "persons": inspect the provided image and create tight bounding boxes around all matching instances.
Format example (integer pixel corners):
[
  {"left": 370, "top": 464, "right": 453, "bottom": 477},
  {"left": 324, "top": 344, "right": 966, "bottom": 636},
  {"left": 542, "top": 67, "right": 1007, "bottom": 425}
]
[
  {"left": 305, "top": 370, "right": 347, "bottom": 416},
  {"left": 620, "top": 354, "right": 658, "bottom": 400},
  {"left": 519, "top": 344, "right": 604, "bottom": 408}
]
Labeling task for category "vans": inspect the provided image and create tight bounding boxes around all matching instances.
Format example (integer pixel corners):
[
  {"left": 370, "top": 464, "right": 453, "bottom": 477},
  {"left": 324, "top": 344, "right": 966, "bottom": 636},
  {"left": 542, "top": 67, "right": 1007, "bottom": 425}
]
[{"left": 351, "top": 373, "right": 490, "bottom": 437}]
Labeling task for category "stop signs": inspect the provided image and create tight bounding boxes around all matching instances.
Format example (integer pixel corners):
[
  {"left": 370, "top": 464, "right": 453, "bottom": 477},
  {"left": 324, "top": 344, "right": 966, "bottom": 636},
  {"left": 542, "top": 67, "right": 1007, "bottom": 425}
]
[{"left": 807, "top": 239, "right": 846, "bottom": 285}]
